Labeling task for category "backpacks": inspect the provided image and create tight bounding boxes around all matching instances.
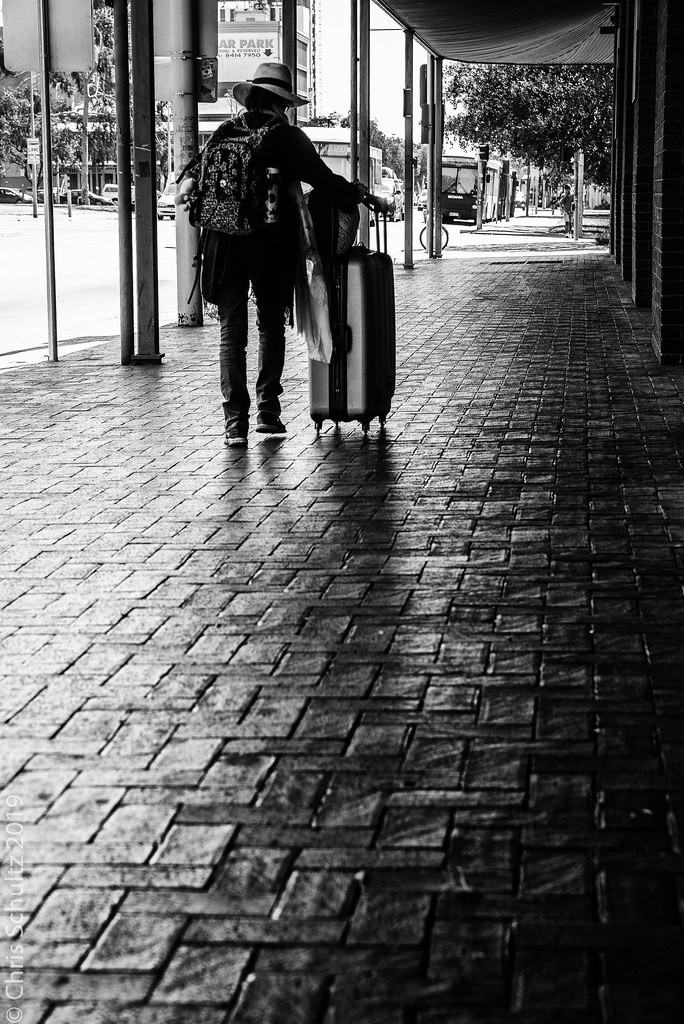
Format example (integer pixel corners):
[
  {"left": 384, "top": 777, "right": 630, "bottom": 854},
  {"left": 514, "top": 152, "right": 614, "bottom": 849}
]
[{"left": 188, "top": 114, "right": 285, "bottom": 236}]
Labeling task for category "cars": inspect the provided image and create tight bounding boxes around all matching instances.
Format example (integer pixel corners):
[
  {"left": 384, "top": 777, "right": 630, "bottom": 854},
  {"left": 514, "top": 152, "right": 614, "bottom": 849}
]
[
  {"left": 514, "top": 191, "right": 526, "bottom": 211},
  {"left": 155, "top": 181, "right": 175, "bottom": 220},
  {"left": 0, "top": 187, "right": 33, "bottom": 204},
  {"left": 381, "top": 165, "right": 427, "bottom": 222},
  {"left": 26, "top": 182, "right": 162, "bottom": 205}
]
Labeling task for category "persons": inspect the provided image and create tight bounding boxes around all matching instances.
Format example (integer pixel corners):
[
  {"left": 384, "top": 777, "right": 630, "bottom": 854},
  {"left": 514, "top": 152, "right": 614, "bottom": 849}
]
[
  {"left": 559, "top": 185, "right": 575, "bottom": 239},
  {"left": 550, "top": 185, "right": 557, "bottom": 218},
  {"left": 175, "top": 63, "right": 371, "bottom": 448}
]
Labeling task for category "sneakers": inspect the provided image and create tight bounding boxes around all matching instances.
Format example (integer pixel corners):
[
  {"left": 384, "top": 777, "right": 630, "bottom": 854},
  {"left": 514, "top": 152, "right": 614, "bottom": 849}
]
[
  {"left": 256, "top": 411, "right": 287, "bottom": 433},
  {"left": 224, "top": 428, "right": 248, "bottom": 447}
]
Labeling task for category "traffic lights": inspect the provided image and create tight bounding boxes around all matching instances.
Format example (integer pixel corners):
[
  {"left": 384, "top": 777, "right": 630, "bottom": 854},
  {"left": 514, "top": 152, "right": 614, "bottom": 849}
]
[{"left": 478, "top": 143, "right": 490, "bottom": 161}]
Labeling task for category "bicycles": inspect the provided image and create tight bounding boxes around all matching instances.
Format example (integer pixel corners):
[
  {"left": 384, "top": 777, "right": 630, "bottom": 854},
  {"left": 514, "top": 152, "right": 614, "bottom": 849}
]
[{"left": 419, "top": 204, "right": 449, "bottom": 253}]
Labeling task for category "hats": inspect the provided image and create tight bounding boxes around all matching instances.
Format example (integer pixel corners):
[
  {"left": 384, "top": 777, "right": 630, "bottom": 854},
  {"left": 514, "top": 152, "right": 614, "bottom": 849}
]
[{"left": 233, "top": 63, "right": 310, "bottom": 108}]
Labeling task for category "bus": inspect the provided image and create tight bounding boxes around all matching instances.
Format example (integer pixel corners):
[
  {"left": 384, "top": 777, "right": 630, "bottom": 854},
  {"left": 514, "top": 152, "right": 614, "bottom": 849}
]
[
  {"left": 299, "top": 125, "right": 383, "bottom": 228},
  {"left": 423, "top": 154, "right": 513, "bottom": 224}
]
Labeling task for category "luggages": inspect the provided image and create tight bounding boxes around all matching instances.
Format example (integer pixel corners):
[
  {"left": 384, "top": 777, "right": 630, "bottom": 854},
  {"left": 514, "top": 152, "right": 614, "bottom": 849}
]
[{"left": 308, "top": 207, "right": 396, "bottom": 432}]
